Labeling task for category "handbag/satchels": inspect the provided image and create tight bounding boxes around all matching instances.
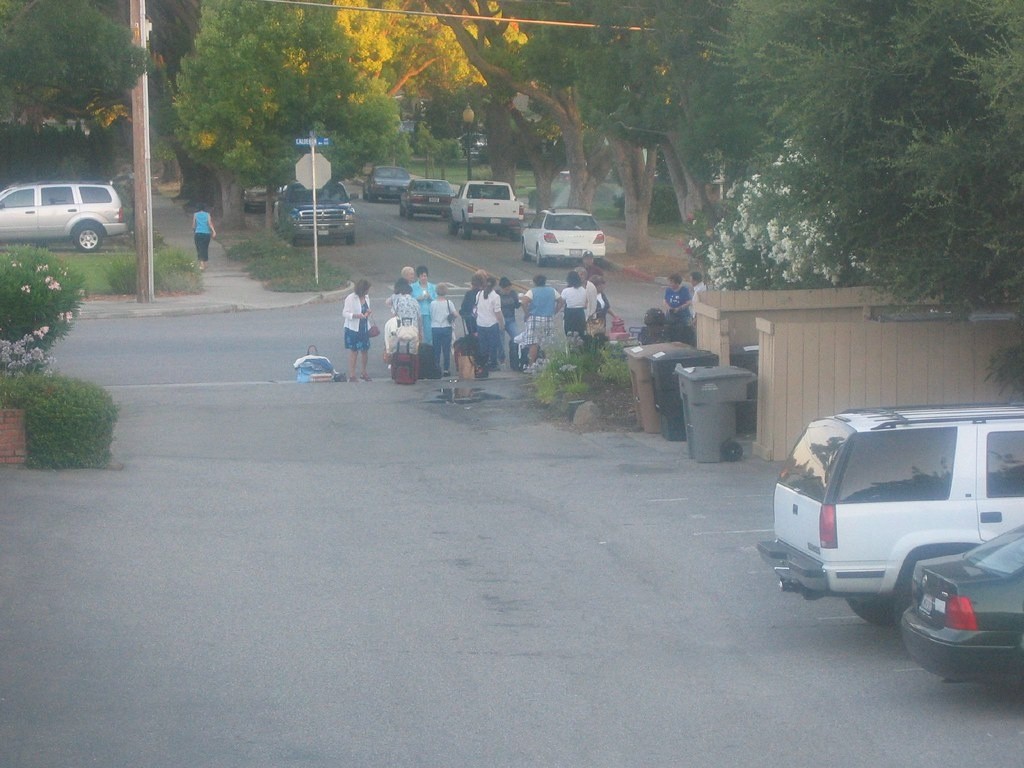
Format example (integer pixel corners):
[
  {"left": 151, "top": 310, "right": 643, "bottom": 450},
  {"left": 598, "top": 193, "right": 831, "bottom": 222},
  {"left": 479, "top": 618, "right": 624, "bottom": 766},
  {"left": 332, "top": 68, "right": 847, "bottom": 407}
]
[
  {"left": 367, "top": 309, "right": 380, "bottom": 337},
  {"left": 457, "top": 348, "right": 477, "bottom": 378},
  {"left": 454, "top": 335, "right": 490, "bottom": 366},
  {"left": 463, "top": 306, "right": 478, "bottom": 333},
  {"left": 585, "top": 310, "right": 607, "bottom": 338},
  {"left": 293, "top": 345, "right": 335, "bottom": 383},
  {"left": 446, "top": 298, "right": 456, "bottom": 322},
  {"left": 638, "top": 306, "right": 693, "bottom": 345},
  {"left": 384, "top": 316, "right": 421, "bottom": 355},
  {"left": 332, "top": 371, "right": 347, "bottom": 382}
]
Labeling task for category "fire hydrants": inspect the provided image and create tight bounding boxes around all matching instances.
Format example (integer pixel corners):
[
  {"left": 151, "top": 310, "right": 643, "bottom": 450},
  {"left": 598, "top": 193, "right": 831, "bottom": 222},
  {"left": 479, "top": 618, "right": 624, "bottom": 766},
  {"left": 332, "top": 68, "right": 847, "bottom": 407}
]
[{"left": 611, "top": 318, "right": 626, "bottom": 333}]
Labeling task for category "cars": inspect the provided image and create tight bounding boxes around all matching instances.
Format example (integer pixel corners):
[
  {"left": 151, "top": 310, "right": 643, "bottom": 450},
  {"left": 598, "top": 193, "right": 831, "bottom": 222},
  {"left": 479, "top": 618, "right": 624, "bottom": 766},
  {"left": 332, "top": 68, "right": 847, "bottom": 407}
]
[
  {"left": 244, "top": 185, "right": 276, "bottom": 213},
  {"left": 400, "top": 178, "right": 456, "bottom": 219},
  {"left": 900, "top": 527, "right": 1024, "bottom": 702},
  {"left": 362, "top": 165, "right": 411, "bottom": 203}
]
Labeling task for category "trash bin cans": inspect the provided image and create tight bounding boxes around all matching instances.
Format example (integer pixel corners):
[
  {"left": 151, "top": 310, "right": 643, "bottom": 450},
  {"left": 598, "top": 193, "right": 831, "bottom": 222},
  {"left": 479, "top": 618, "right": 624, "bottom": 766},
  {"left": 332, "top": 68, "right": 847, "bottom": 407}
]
[
  {"left": 646, "top": 348, "right": 720, "bottom": 441},
  {"left": 676, "top": 363, "right": 757, "bottom": 463},
  {"left": 623, "top": 341, "right": 694, "bottom": 434}
]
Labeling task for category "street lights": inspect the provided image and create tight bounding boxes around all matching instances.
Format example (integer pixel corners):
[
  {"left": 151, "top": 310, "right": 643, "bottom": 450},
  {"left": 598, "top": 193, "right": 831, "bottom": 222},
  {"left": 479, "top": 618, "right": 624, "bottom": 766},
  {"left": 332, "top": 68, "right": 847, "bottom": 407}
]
[{"left": 462, "top": 101, "right": 475, "bottom": 180}]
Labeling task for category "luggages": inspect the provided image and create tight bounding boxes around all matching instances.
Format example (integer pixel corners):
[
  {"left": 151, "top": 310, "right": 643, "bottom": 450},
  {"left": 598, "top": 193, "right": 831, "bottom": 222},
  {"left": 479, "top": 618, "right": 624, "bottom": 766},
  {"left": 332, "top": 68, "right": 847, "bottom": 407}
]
[
  {"left": 417, "top": 343, "right": 435, "bottom": 378},
  {"left": 391, "top": 340, "right": 417, "bottom": 384},
  {"left": 509, "top": 337, "right": 545, "bottom": 372},
  {"left": 609, "top": 318, "right": 631, "bottom": 340}
]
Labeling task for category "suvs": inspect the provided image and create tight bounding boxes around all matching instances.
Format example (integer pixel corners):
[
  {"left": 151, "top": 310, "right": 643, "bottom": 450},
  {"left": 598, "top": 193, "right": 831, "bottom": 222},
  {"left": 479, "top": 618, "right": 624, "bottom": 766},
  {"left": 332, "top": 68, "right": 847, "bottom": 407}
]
[
  {"left": 756, "top": 405, "right": 1024, "bottom": 625},
  {"left": 0, "top": 180, "right": 128, "bottom": 253},
  {"left": 521, "top": 208, "right": 605, "bottom": 268}
]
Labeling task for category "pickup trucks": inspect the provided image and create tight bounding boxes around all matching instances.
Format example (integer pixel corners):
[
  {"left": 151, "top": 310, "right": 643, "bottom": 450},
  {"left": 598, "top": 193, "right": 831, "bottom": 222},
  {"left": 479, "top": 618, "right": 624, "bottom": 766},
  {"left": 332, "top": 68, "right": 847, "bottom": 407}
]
[
  {"left": 448, "top": 179, "right": 524, "bottom": 240},
  {"left": 278, "top": 182, "right": 356, "bottom": 247}
]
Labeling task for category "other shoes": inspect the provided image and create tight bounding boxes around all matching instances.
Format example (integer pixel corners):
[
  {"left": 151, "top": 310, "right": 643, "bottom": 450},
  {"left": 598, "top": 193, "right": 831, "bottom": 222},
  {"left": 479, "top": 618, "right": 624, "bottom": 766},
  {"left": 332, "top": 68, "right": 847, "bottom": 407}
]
[
  {"left": 349, "top": 376, "right": 359, "bottom": 382},
  {"left": 360, "top": 372, "right": 372, "bottom": 381},
  {"left": 501, "top": 360, "right": 507, "bottom": 366}
]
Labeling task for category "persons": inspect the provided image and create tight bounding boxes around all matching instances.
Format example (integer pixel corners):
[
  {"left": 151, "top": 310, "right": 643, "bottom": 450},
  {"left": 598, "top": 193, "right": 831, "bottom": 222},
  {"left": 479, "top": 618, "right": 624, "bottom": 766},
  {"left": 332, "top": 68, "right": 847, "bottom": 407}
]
[
  {"left": 689, "top": 271, "right": 708, "bottom": 332},
  {"left": 384, "top": 250, "right": 621, "bottom": 387},
  {"left": 192, "top": 202, "right": 216, "bottom": 270},
  {"left": 663, "top": 272, "right": 692, "bottom": 322},
  {"left": 342, "top": 279, "right": 373, "bottom": 383}
]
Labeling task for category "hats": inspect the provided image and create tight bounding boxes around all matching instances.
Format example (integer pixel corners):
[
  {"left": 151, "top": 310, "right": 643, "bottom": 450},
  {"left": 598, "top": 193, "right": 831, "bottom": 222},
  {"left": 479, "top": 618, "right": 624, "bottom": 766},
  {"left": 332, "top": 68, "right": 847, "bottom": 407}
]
[
  {"left": 583, "top": 251, "right": 593, "bottom": 257},
  {"left": 498, "top": 277, "right": 512, "bottom": 288}
]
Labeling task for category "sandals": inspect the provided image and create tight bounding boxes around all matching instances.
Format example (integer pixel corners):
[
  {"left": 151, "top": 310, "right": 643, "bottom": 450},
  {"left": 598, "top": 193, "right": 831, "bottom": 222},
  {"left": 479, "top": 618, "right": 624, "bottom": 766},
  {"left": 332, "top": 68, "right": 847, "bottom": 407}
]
[{"left": 443, "top": 368, "right": 450, "bottom": 376}]
